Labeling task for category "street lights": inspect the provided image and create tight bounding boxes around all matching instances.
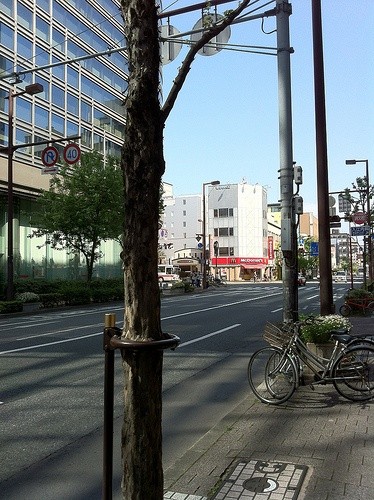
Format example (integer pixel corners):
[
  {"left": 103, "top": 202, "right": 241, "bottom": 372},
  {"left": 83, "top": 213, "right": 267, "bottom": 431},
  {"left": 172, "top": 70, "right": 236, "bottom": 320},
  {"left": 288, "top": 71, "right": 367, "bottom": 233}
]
[
  {"left": 345, "top": 159, "right": 373, "bottom": 279},
  {"left": 3, "top": 83, "right": 43, "bottom": 302},
  {"left": 202, "top": 180, "right": 221, "bottom": 290}
]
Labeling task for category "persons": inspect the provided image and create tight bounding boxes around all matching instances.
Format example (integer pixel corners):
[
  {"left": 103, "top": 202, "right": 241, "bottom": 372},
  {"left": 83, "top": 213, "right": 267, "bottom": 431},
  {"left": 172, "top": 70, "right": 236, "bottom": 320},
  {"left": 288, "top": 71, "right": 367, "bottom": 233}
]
[
  {"left": 191, "top": 273, "right": 201, "bottom": 288},
  {"left": 211, "top": 272, "right": 220, "bottom": 281},
  {"left": 253, "top": 271, "right": 256, "bottom": 283},
  {"left": 206, "top": 274, "right": 210, "bottom": 288},
  {"left": 264, "top": 272, "right": 267, "bottom": 281}
]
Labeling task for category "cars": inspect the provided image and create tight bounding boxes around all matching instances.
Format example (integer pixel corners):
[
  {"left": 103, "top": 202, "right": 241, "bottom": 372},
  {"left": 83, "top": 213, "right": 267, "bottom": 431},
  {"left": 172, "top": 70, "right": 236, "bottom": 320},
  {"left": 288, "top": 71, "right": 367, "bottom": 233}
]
[
  {"left": 335, "top": 271, "right": 348, "bottom": 283},
  {"left": 356, "top": 268, "right": 368, "bottom": 277},
  {"left": 298, "top": 273, "right": 307, "bottom": 286}
]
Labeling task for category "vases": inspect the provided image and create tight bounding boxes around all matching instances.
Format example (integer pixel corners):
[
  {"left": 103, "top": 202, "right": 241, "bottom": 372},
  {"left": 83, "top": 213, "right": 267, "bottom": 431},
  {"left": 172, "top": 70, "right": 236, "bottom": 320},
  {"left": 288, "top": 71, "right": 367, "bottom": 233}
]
[
  {"left": 306, "top": 343, "right": 343, "bottom": 372},
  {"left": 22, "top": 303, "right": 38, "bottom": 312}
]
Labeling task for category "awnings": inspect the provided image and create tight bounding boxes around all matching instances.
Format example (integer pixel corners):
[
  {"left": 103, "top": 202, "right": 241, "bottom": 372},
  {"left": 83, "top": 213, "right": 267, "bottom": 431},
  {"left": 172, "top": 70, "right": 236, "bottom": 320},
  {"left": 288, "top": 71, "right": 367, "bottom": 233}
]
[{"left": 241, "top": 264, "right": 265, "bottom": 270}]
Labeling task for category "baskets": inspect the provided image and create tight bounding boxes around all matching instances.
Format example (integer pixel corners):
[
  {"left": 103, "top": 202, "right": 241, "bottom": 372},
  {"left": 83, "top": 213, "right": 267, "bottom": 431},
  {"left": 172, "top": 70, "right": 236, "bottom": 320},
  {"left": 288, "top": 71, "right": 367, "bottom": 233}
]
[{"left": 263, "top": 319, "right": 292, "bottom": 348}]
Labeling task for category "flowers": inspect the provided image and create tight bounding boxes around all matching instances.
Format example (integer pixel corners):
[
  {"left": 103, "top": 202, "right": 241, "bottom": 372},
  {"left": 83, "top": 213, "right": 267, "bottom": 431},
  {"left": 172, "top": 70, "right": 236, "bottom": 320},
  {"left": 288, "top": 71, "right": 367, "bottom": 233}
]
[
  {"left": 16, "top": 291, "right": 40, "bottom": 304},
  {"left": 298, "top": 314, "right": 353, "bottom": 343}
]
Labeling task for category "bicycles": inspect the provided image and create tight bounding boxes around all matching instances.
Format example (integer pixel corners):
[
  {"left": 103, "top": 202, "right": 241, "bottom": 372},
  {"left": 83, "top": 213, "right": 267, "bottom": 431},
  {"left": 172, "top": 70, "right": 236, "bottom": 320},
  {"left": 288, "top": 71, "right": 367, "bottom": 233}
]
[
  {"left": 339, "top": 297, "right": 374, "bottom": 317},
  {"left": 247, "top": 315, "right": 374, "bottom": 405}
]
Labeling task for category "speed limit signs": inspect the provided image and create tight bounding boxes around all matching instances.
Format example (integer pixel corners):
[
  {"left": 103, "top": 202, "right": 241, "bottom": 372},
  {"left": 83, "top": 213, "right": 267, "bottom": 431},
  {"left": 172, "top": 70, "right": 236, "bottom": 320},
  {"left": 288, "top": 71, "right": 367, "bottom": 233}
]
[{"left": 63, "top": 144, "right": 81, "bottom": 164}]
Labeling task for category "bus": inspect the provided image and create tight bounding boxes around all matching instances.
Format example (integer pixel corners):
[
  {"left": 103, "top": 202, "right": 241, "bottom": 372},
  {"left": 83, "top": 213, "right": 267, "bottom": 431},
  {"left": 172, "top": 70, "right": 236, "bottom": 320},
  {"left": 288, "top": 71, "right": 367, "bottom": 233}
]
[{"left": 157, "top": 262, "right": 198, "bottom": 282}]
